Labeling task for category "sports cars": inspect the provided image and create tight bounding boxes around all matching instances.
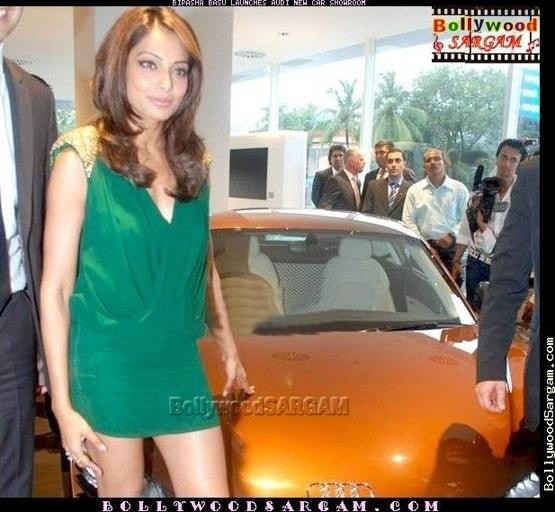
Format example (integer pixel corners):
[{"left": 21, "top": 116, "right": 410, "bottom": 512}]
[{"left": 140, "top": 203, "right": 543, "bottom": 502}]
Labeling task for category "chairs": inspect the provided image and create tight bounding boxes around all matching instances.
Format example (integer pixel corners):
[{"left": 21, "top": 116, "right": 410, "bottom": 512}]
[
  {"left": 203, "top": 234, "right": 284, "bottom": 337},
  {"left": 317, "top": 235, "right": 397, "bottom": 314}
]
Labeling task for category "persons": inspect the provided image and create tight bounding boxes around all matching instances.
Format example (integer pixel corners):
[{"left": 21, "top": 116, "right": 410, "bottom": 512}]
[
  {"left": 0, "top": 6, "right": 257, "bottom": 499},
  {"left": 311, "top": 6, "right": 540, "bottom": 499}
]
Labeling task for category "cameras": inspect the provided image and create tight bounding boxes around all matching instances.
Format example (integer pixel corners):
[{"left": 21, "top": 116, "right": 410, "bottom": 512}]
[{"left": 525, "top": 137, "right": 533, "bottom": 146}]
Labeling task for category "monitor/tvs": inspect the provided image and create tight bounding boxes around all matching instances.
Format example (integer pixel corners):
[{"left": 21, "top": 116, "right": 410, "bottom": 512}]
[{"left": 229, "top": 145, "right": 269, "bottom": 202}]
